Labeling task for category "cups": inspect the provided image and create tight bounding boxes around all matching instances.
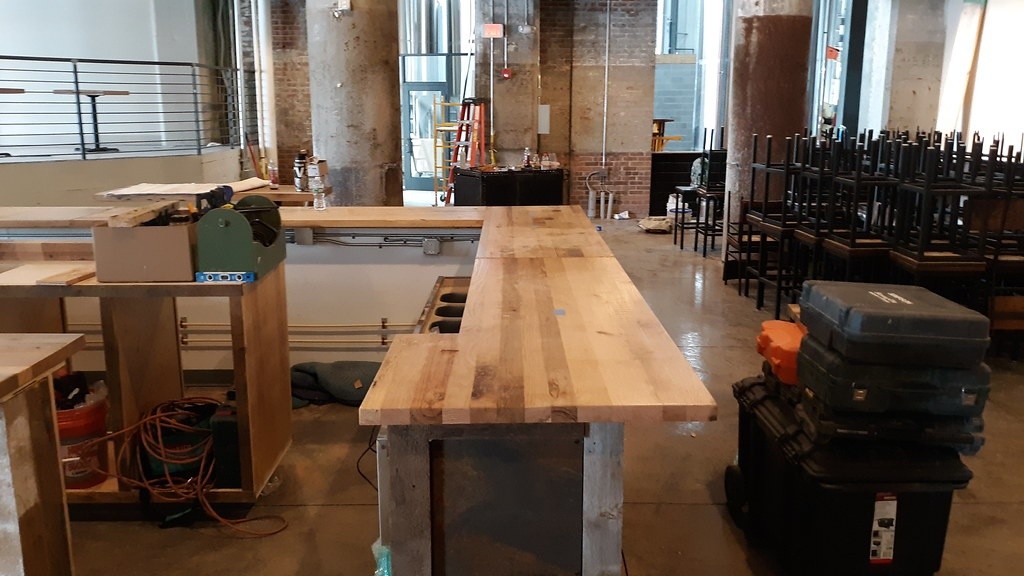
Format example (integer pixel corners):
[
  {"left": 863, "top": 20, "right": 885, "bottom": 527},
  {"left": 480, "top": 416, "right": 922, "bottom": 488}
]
[
  {"left": 550, "top": 162, "right": 560, "bottom": 170},
  {"left": 541, "top": 161, "right": 550, "bottom": 170}
]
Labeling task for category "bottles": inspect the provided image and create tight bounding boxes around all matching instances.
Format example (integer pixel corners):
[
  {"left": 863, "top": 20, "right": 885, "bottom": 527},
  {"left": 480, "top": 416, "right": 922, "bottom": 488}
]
[
  {"left": 293, "top": 149, "right": 320, "bottom": 192},
  {"left": 312, "top": 177, "right": 326, "bottom": 210},
  {"left": 524, "top": 147, "right": 560, "bottom": 170},
  {"left": 268, "top": 159, "right": 279, "bottom": 189}
]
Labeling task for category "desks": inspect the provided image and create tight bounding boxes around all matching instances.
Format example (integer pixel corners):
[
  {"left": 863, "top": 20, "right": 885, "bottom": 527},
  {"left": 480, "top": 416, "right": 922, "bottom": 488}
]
[
  {"left": 0, "top": 260, "right": 293, "bottom": 505},
  {"left": 232, "top": 185, "right": 334, "bottom": 207}
]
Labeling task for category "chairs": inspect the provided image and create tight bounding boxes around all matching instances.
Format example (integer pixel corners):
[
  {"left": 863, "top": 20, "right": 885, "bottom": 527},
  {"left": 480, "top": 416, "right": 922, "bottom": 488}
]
[{"left": 672, "top": 128, "right": 1024, "bottom": 323}]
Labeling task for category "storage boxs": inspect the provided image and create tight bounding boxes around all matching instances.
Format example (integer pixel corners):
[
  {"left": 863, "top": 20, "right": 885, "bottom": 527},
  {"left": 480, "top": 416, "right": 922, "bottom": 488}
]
[
  {"left": 731, "top": 280, "right": 992, "bottom": 576},
  {"left": 309, "top": 159, "right": 328, "bottom": 190},
  {"left": 90, "top": 220, "right": 199, "bottom": 282}
]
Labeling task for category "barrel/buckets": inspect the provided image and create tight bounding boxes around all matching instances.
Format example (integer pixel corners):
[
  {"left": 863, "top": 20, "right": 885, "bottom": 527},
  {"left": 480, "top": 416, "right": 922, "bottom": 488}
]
[
  {"left": 666, "top": 203, "right": 692, "bottom": 230},
  {"left": 52, "top": 371, "right": 109, "bottom": 488}
]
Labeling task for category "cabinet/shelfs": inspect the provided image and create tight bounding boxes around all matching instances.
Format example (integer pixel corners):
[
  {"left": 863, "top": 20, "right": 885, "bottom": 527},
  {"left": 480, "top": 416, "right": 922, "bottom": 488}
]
[{"left": 453, "top": 166, "right": 564, "bottom": 207}]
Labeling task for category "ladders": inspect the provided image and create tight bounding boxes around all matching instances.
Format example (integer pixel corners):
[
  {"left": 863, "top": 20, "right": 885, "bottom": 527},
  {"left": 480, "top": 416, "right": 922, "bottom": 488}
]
[{"left": 444, "top": 97, "right": 489, "bottom": 206}]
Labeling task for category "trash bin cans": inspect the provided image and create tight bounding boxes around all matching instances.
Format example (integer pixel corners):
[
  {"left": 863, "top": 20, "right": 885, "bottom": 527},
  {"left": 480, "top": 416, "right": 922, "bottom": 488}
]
[{"left": 677, "top": 186, "right": 699, "bottom": 220}]
